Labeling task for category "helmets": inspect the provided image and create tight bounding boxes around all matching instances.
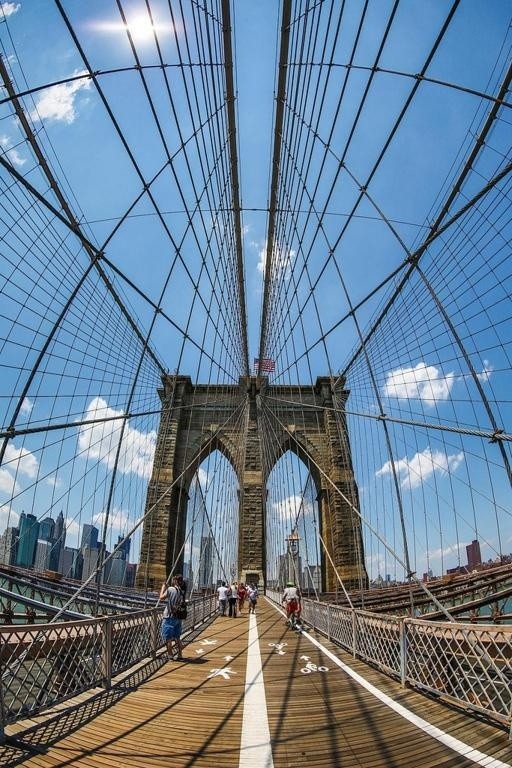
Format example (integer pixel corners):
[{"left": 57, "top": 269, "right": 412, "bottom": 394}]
[{"left": 287, "top": 582, "right": 294, "bottom": 586}]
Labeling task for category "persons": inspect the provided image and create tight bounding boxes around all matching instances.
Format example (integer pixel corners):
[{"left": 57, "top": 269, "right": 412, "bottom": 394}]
[
  {"left": 234, "top": 581, "right": 259, "bottom": 615},
  {"left": 226, "top": 583, "right": 238, "bottom": 618},
  {"left": 281, "top": 581, "right": 301, "bottom": 627},
  {"left": 215, "top": 581, "right": 229, "bottom": 618},
  {"left": 159, "top": 573, "right": 186, "bottom": 662}
]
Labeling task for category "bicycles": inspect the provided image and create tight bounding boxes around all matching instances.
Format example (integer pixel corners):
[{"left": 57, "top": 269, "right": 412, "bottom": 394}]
[{"left": 282, "top": 599, "right": 301, "bottom": 631}]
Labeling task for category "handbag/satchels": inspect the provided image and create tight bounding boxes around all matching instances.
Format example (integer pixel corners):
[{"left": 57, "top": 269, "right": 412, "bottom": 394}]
[{"left": 250, "top": 597, "right": 257, "bottom": 604}]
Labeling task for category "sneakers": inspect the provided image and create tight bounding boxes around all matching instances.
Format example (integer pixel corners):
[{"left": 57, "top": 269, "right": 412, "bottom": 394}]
[
  {"left": 162, "top": 655, "right": 173, "bottom": 662},
  {"left": 174, "top": 656, "right": 184, "bottom": 661},
  {"left": 239, "top": 609, "right": 257, "bottom": 615}
]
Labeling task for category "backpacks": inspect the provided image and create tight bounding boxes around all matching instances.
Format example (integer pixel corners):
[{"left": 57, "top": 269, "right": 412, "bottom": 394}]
[{"left": 173, "top": 595, "right": 187, "bottom": 619}]
